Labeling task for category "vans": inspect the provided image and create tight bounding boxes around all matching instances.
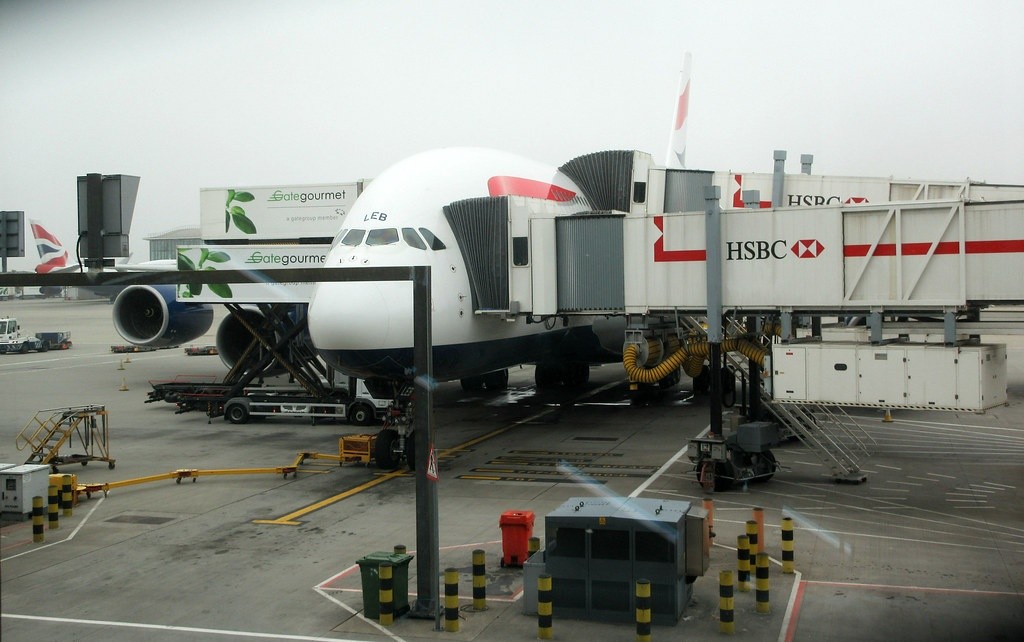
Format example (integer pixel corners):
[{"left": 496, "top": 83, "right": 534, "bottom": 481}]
[{"left": 0, "top": 318, "right": 17, "bottom": 343}]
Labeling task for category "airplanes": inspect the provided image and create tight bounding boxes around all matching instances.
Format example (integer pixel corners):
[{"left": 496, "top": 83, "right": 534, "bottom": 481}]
[{"left": 0, "top": 145, "right": 1023, "bottom": 493}]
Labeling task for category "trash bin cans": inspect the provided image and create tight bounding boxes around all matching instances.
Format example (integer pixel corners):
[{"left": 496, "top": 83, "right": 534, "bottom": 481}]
[
  {"left": 356, "top": 551, "right": 415, "bottom": 619},
  {"left": 499, "top": 510, "right": 536, "bottom": 568}
]
